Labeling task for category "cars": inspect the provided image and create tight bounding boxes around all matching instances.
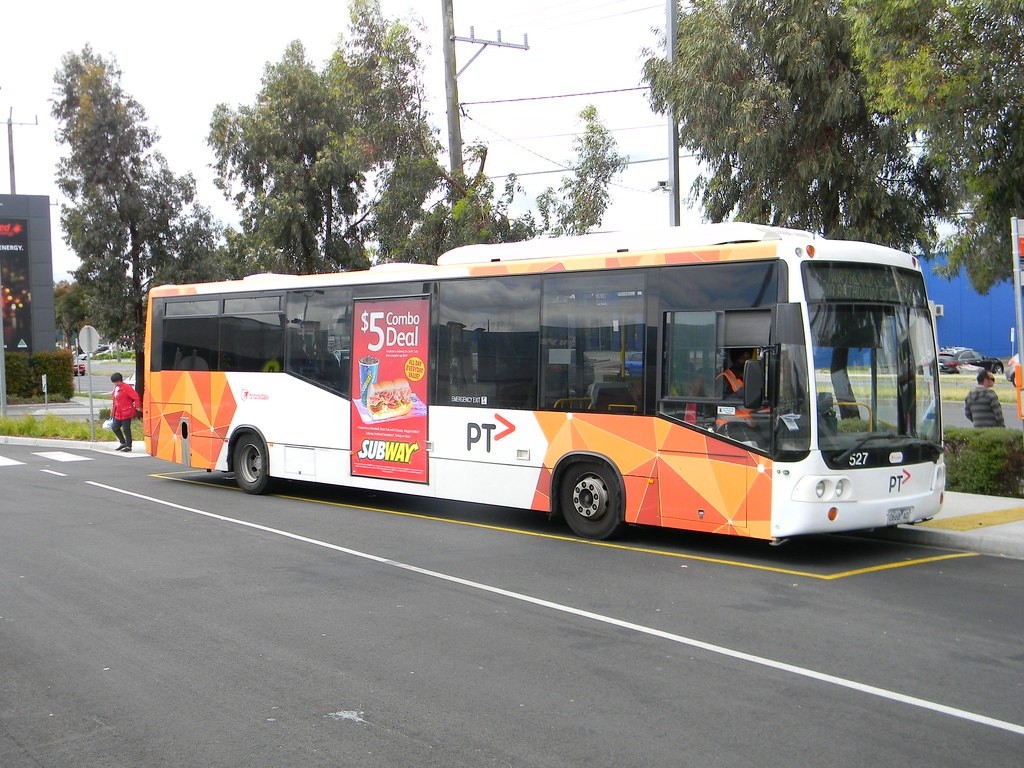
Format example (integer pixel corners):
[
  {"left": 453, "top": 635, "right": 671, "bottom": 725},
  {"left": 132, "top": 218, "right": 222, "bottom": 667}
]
[
  {"left": 1006, "top": 353, "right": 1019, "bottom": 387},
  {"left": 71, "top": 360, "right": 85, "bottom": 376}
]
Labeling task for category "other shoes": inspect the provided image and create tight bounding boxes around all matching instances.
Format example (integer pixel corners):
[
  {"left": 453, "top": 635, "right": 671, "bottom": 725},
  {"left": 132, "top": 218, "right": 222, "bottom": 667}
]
[{"left": 116, "top": 445, "right": 132, "bottom": 452}]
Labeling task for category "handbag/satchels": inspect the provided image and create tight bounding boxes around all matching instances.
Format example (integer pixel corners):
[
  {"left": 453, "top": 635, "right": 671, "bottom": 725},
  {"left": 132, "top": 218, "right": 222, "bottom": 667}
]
[{"left": 102, "top": 417, "right": 122, "bottom": 431}]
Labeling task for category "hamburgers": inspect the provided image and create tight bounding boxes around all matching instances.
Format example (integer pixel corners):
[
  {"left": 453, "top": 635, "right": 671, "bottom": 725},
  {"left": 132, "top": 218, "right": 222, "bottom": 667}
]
[{"left": 367, "top": 378, "right": 412, "bottom": 421}]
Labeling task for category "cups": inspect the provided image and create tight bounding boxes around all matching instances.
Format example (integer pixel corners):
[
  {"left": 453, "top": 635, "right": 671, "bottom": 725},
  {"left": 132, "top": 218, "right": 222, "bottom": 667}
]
[{"left": 358, "top": 360, "right": 379, "bottom": 407}]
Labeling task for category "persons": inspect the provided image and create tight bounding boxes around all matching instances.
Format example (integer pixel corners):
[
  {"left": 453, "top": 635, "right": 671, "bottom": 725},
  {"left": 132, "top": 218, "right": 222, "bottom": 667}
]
[
  {"left": 109, "top": 374, "right": 142, "bottom": 456},
  {"left": 964, "top": 370, "right": 1006, "bottom": 428},
  {"left": 715, "top": 347, "right": 770, "bottom": 449}
]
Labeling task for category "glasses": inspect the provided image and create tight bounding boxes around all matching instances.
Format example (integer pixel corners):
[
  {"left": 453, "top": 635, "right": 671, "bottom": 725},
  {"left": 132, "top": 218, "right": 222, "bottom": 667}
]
[{"left": 989, "top": 378, "right": 995, "bottom": 382}]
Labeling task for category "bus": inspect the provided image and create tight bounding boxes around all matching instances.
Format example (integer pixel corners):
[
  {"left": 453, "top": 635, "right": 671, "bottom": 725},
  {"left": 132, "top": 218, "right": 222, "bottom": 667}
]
[{"left": 142, "top": 222, "right": 946, "bottom": 541}]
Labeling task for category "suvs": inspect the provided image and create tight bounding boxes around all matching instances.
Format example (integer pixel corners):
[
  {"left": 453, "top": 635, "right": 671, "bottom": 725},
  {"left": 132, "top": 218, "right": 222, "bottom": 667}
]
[{"left": 938, "top": 346, "right": 1004, "bottom": 374}]
